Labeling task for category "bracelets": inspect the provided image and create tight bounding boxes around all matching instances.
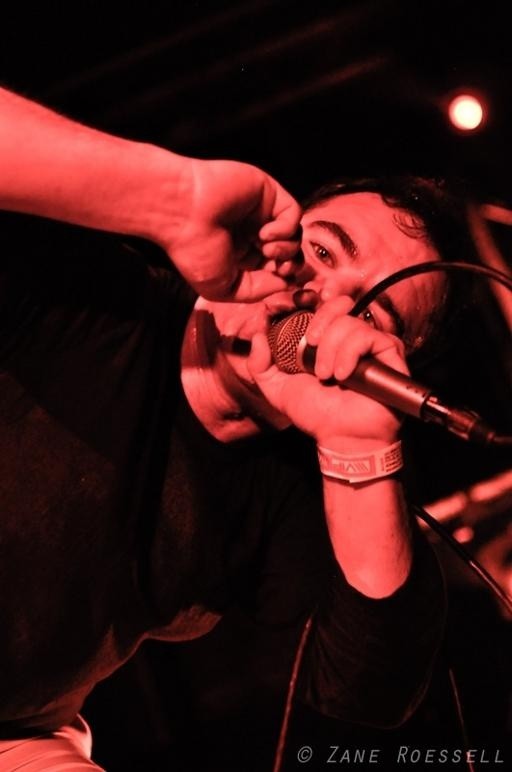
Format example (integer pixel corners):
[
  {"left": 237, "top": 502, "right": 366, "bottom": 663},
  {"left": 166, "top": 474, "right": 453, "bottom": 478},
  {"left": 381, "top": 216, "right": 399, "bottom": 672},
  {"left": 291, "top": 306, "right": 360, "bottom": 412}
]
[{"left": 316, "top": 440, "right": 407, "bottom": 484}]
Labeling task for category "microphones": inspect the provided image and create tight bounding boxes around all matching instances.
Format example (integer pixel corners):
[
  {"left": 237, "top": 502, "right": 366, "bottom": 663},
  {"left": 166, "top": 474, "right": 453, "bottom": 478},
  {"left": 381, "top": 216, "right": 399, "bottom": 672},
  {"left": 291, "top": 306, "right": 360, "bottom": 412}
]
[{"left": 261, "top": 306, "right": 511, "bottom": 458}]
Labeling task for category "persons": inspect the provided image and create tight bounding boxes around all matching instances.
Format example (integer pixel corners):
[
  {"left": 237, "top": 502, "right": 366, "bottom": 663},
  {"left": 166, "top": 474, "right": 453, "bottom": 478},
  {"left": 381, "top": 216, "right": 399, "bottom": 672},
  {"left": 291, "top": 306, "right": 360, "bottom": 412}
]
[{"left": 1, "top": 87, "right": 479, "bottom": 771}]
